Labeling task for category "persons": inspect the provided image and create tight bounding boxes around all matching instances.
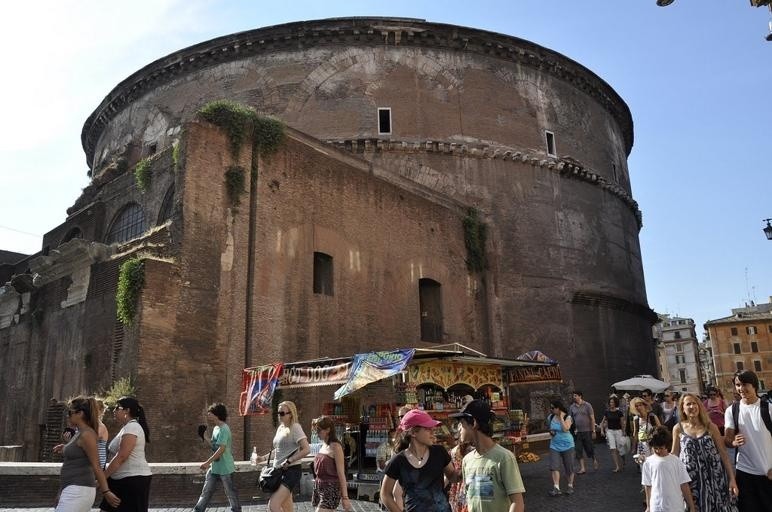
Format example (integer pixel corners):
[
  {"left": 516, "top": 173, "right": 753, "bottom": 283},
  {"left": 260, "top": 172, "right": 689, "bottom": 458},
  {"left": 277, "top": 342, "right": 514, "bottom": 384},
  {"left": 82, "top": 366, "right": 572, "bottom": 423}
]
[
  {"left": 624, "top": 387, "right": 740, "bottom": 512},
  {"left": 391, "top": 406, "right": 411, "bottom": 511},
  {"left": 599, "top": 396, "right": 627, "bottom": 472},
  {"left": 52, "top": 398, "right": 109, "bottom": 493},
  {"left": 722, "top": 369, "right": 772, "bottom": 512},
  {"left": 728, "top": 387, "right": 742, "bottom": 464},
  {"left": 447, "top": 399, "right": 526, "bottom": 511},
  {"left": 192, "top": 402, "right": 243, "bottom": 512},
  {"left": 546, "top": 400, "right": 576, "bottom": 496},
  {"left": 255, "top": 400, "right": 311, "bottom": 511},
  {"left": 450, "top": 423, "right": 475, "bottom": 511},
  {"left": 308, "top": 414, "right": 355, "bottom": 512},
  {"left": 570, "top": 392, "right": 599, "bottom": 474},
  {"left": 93, "top": 395, "right": 153, "bottom": 511},
  {"left": 380, "top": 410, "right": 463, "bottom": 511},
  {"left": 55, "top": 394, "right": 122, "bottom": 512}
]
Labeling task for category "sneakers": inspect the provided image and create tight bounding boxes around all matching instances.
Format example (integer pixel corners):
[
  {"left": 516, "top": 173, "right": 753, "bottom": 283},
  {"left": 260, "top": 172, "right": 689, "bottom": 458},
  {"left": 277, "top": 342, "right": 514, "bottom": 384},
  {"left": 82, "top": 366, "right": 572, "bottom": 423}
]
[
  {"left": 565, "top": 487, "right": 576, "bottom": 495},
  {"left": 548, "top": 488, "right": 562, "bottom": 496}
]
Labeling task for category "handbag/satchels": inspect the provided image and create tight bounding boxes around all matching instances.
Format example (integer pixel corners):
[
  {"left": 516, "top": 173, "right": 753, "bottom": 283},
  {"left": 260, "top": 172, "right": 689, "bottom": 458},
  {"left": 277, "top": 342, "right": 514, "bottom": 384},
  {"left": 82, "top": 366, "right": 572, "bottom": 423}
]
[
  {"left": 257, "top": 465, "right": 285, "bottom": 494},
  {"left": 664, "top": 407, "right": 678, "bottom": 431}
]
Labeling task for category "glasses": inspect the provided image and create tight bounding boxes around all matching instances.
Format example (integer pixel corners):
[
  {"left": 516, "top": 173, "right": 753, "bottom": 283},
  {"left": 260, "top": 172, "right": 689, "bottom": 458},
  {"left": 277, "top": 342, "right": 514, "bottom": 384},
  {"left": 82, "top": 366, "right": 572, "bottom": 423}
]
[
  {"left": 397, "top": 415, "right": 404, "bottom": 420},
  {"left": 279, "top": 411, "right": 291, "bottom": 417},
  {"left": 68, "top": 410, "right": 78, "bottom": 417},
  {"left": 664, "top": 395, "right": 672, "bottom": 397},
  {"left": 316, "top": 428, "right": 324, "bottom": 433},
  {"left": 112, "top": 405, "right": 125, "bottom": 411},
  {"left": 550, "top": 406, "right": 556, "bottom": 410},
  {"left": 642, "top": 395, "right": 649, "bottom": 397}
]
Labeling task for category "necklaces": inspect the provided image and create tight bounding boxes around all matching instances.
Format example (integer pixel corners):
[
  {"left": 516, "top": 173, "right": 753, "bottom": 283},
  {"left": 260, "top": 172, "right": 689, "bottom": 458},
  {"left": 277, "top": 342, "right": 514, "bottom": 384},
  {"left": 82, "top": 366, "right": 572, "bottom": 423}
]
[{"left": 409, "top": 447, "right": 426, "bottom": 465}]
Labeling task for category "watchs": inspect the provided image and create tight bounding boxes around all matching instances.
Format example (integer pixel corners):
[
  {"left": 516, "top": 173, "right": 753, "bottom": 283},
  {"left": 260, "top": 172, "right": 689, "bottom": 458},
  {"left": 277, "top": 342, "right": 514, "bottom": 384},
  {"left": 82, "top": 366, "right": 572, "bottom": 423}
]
[{"left": 592, "top": 431, "right": 595, "bottom": 433}]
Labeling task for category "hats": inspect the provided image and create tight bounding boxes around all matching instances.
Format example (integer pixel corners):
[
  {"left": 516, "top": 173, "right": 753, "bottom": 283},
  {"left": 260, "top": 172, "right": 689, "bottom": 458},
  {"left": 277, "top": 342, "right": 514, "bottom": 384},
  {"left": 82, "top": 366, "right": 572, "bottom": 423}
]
[
  {"left": 630, "top": 397, "right": 653, "bottom": 416},
  {"left": 448, "top": 399, "right": 491, "bottom": 418},
  {"left": 400, "top": 410, "right": 442, "bottom": 431}
]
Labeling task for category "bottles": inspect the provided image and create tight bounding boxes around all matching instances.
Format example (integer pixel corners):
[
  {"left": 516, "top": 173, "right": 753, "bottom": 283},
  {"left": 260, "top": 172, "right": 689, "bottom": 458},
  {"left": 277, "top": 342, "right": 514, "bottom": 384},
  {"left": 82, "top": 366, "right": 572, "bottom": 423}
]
[
  {"left": 250, "top": 446, "right": 258, "bottom": 467},
  {"left": 424, "top": 388, "right": 468, "bottom": 410},
  {"left": 365, "top": 429, "right": 389, "bottom": 458}
]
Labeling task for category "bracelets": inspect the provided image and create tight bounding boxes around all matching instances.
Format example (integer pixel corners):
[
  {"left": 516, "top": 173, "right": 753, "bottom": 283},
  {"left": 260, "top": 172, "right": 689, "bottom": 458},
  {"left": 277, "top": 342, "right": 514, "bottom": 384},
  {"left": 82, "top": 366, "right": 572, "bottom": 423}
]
[
  {"left": 341, "top": 495, "right": 349, "bottom": 500},
  {"left": 284, "top": 459, "right": 292, "bottom": 465},
  {"left": 101, "top": 490, "right": 111, "bottom": 495}
]
